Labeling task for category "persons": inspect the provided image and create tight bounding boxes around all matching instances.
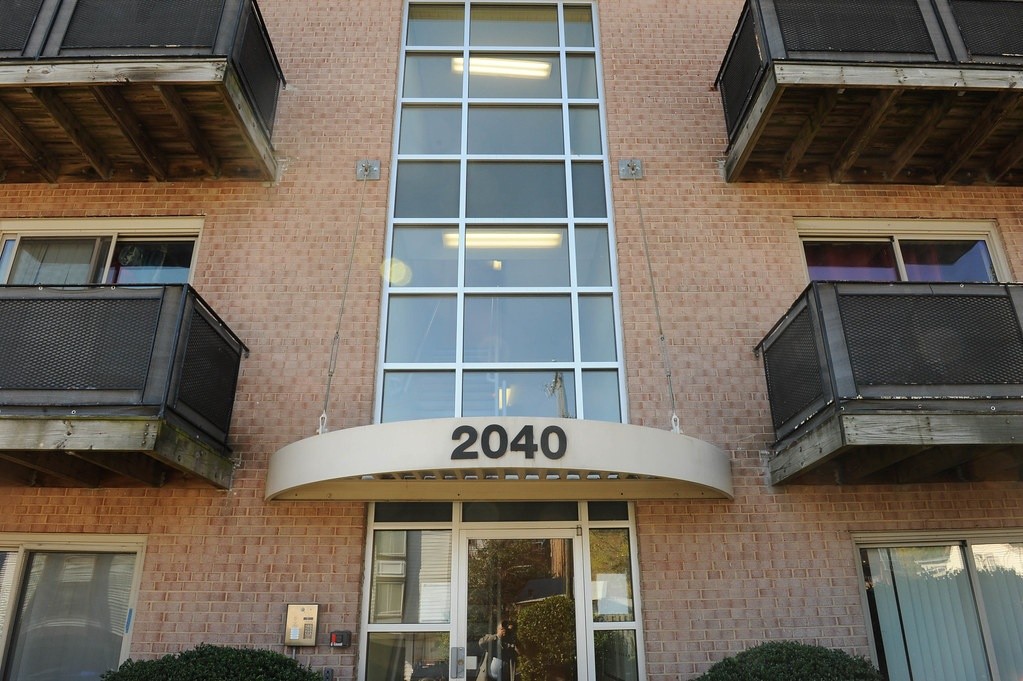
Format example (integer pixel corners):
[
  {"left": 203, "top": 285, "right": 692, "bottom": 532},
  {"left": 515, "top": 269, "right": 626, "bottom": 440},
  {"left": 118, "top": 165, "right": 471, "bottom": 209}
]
[{"left": 475, "top": 620, "right": 524, "bottom": 681}]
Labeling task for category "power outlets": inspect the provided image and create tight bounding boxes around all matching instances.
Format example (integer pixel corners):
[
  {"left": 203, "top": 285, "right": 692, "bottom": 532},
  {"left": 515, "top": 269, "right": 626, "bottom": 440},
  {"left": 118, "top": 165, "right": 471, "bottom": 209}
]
[
  {"left": 356, "top": 160, "right": 381, "bottom": 180},
  {"left": 619, "top": 160, "right": 642, "bottom": 180}
]
